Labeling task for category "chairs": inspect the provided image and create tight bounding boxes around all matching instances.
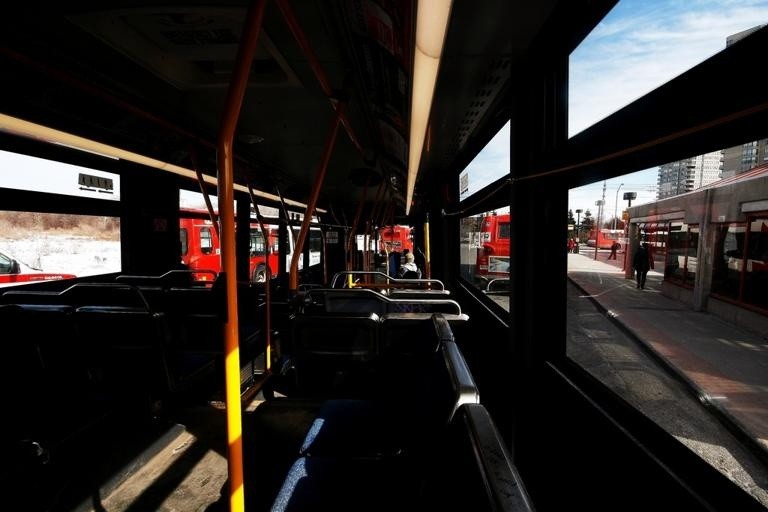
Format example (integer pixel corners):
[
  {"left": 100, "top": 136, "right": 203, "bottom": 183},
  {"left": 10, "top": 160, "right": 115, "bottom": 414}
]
[{"left": 0, "top": 267, "right": 537, "bottom": 512}]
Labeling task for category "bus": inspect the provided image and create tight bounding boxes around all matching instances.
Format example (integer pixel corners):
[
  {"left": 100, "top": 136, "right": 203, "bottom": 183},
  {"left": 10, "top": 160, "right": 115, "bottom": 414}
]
[
  {"left": 640, "top": 215, "right": 768, "bottom": 283},
  {"left": 568, "top": 223, "right": 579, "bottom": 252},
  {"left": 379, "top": 221, "right": 416, "bottom": 257},
  {"left": 587, "top": 229, "right": 627, "bottom": 252},
  {"left": 474, "top": 209, "right": 509, "bottom": 289},
  {"left": 178, "top": 204, "right": 324, "bottom": 287}
]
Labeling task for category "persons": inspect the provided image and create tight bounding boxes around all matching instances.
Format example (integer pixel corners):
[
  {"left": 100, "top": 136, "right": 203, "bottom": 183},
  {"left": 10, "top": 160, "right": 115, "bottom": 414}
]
[
  {"left": 398, "top": 252, "right": 423, "bottom": 280},
  {"left": 568, "top": 238, "right": 576, "bottom": 254},
  {"left": 607, "top": 241, "right": 620, "bottom": 260},
  {"left": 630, "top": 243, "right": 656, "bottom": 291}
]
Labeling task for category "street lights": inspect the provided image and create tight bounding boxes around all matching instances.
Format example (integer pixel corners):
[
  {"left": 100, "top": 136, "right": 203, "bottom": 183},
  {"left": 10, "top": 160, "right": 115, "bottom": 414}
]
[
  {"left": 614, "top": 183, "right": 625, "bottom": 230},
  {"left": 623, "top": 191, "right": 640, "bottom": 273},
  {"left": 576, "top": 209, "right": 583, "bottom": 254}
]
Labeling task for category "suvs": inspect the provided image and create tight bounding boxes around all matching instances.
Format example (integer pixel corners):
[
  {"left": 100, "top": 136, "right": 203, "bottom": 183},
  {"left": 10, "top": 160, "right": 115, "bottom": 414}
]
[{"left": 0, "top": 248, "right": 78, "bottom": 294}]
